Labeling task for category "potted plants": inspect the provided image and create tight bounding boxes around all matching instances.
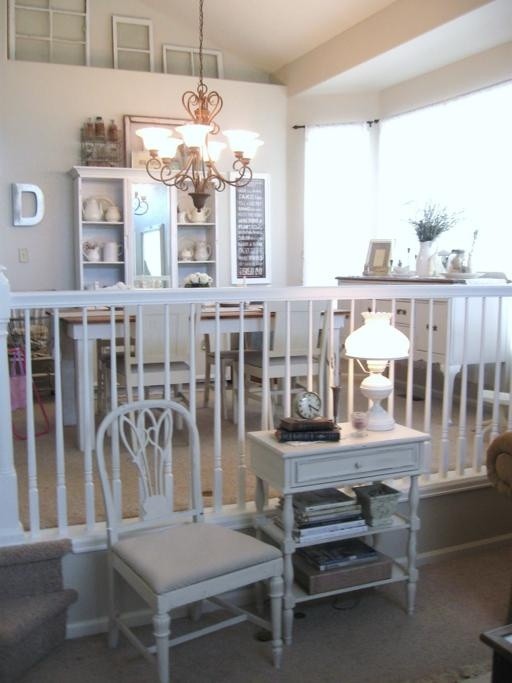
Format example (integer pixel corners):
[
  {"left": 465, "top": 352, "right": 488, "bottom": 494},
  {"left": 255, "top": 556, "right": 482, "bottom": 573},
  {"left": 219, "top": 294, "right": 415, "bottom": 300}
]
[{"left": 402, "top": 202, "right": 464, "bottom": 277}]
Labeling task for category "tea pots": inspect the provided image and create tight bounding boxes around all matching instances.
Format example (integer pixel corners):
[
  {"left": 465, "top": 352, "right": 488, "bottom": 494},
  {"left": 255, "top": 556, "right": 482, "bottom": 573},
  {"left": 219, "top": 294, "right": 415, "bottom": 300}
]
[
  {"left": 102, "top": 241, "right": 123, "bottom": 262},
  {"left": 82, "top": 196, "right": 103, "bottom": 221},
  {"left": 83, "top": 247, "right": 101, "bottom": 261},
  {"left": 187, "top": 205, "right": 210, "bottom": 223},
  {"left": 192, "top": 240, "right": 213, "bottom": 261}
]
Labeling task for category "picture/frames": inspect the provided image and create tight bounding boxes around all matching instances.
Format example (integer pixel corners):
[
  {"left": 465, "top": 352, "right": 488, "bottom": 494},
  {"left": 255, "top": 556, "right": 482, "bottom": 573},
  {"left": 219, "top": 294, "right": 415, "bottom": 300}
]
[
  {"left": 364, "top": 238, "right": 395, "bottom": 276},
  {"left": 123, "top": 114, "right": 204, "bottom": 169}
]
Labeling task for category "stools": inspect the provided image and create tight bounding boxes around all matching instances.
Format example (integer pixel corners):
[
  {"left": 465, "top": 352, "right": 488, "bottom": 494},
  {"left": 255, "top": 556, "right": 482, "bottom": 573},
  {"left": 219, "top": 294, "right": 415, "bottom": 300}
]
[{"left": 472, "top": 390, "right": 511, "bottom": 440}]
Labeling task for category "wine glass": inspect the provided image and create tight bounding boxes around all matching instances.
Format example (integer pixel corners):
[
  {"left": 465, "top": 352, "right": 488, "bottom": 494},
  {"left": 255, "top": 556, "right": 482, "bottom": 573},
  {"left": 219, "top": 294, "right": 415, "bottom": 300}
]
[{"left": 351, "top": 412, "right": 369, "bottom": 439}]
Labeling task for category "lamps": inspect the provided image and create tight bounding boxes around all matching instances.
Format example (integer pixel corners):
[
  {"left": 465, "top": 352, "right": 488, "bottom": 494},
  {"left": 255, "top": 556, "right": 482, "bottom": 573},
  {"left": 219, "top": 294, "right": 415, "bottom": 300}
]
[
  {"left": 344, "top": 310, "right": 409, "bottom": 431},
  {"left": 135, "top": 0, "right": 264, "bottom": 212},
  {"left": 133, "top": 184, "right": 155, "bottom": 215}
]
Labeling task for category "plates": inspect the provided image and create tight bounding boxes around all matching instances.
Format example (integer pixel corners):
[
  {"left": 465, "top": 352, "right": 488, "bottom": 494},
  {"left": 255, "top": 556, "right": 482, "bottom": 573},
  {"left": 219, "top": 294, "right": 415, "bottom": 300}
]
[{"left": 440, "top": 273, "right": 485, "bottom": 278}]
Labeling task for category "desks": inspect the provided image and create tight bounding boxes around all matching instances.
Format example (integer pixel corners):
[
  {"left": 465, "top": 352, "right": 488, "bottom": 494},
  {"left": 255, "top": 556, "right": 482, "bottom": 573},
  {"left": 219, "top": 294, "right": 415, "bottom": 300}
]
[
  {"left": 479, "top": 625, "right": 512, "bottom": 683},
  {"left": 45, "top": 304, "right": 349, "bottom": 452}
]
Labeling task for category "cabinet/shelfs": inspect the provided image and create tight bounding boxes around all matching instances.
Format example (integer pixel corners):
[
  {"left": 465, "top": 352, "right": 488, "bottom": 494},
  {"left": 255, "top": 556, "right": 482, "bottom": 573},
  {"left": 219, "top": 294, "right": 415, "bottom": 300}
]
[
  {"left": 334, "top": 276, "right": 509, "bottom": 426},
  {"left": 67, "top": 166, "right": 127, "bottom": 290},
  {"left": 80, "top": 128, "right": 121, "bottom": 167},
  {"left": 247, "top": 421, "right": 431, "bottom": 646},
  {"left": 170, "top": 170, "right": 219, "bottom": 289}
]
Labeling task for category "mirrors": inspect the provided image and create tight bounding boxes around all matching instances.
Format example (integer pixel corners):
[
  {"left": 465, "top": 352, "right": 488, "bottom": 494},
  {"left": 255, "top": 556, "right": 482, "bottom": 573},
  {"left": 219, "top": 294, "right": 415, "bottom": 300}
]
[{"left": 128, "top": 168, "right": 175, "bottom": 289}]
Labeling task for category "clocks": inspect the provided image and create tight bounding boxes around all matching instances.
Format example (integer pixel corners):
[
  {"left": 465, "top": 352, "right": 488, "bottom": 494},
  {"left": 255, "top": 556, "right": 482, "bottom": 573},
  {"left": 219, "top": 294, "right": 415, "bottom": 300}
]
[{"left": 295, "top": 392, "right": 322, "bottom": 419}]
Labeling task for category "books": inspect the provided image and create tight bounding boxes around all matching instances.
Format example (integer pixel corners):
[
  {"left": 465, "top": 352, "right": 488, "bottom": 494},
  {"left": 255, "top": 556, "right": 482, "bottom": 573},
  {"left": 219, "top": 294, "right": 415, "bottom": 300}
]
[
  {"left": 298, "top": 538, "right": 381, "bottom": 571},
  {"left": 272, "top": 486, "right": 368, "bottom": 543},
  {"left": 274, "top": 415, "right": 341, "bottom": 442}
]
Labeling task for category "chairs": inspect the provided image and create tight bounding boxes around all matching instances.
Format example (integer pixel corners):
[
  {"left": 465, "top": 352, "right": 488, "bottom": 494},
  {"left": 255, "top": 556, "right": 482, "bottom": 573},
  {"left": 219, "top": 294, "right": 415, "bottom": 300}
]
[
  {"left": 96, "top": 301, "right": 333, "bottom": 439},
  {"left": 97, "top": 399, "right": 284, "bottom": 683}
]
